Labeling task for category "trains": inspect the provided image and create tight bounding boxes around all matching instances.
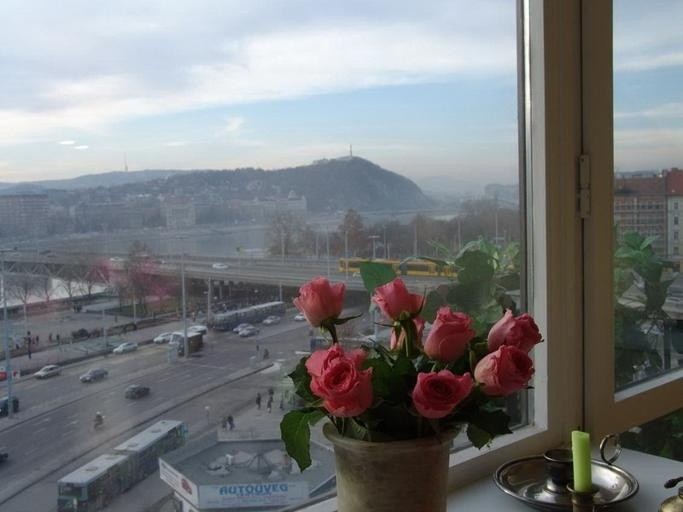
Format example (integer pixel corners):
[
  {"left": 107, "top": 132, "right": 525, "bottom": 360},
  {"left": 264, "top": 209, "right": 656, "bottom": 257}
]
[{"left": 338, "top": 257, "right": 461, "bottom": 278}]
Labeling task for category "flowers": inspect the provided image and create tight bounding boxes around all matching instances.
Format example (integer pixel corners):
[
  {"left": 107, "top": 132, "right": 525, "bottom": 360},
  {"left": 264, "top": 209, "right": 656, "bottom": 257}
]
[{"left": 279, "top": 265, "right": 543, "bottom": 472}]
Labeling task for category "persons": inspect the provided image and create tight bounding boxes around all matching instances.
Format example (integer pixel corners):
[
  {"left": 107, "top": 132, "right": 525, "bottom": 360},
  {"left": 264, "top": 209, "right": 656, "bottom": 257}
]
[
  {"left": 226, "top": 415, "right": 235, "bottom": 431},
  {"left": 262, "top": 348, "right": 268, "bottom": 359},
  {"left": 221, "top": 416, "right": 226, "bottom": 431},
  {"left": 266, "top": 400, "right": 271, "bottom": 414},
  {"left": 254, "top": 392, "right": 261, "bottom": 409},
  {"left": 95, "top": 411, "right": 102, "bottom": 422},
  {"left": 267, "top": 388, "right": 274, "bottom": 402}
]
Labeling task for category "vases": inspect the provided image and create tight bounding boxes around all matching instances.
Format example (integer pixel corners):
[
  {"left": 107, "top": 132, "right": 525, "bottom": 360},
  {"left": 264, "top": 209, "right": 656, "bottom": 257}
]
[{"left": 321, "top": 421, "right": 461, "bottom": 510}]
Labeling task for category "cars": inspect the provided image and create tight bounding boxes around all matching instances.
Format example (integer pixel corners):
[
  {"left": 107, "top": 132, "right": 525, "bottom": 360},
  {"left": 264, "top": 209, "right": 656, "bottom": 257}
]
[
  {"left": 124, "top": 382, "right": 150, "bottom": 400},
  {"left": 211, "top": 262, "right": 228, "bottom": 271},
  {"left": 78, "top": 366, "right": 108, "bottom": 385},
  {"left": 153, "top": 324, "right": 208, "bottom": 358},
  {"left": 31, "top": 363, "right": 64, "bottom": 379},
  {"left": 214, "top": 299, "right": 307, "bottom": 337},
  {"left": 351, "top": 272, "right": 361, "bottom": 280},
  {"left": 109, "top": 256, "right": 123, "bottom": 262},
  {"left": 112, "top": 340, "right": 138, "bottom": 355}
]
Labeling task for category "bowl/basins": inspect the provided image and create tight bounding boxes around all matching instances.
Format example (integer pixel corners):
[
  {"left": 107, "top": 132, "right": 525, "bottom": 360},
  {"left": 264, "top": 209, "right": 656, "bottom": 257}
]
[
  {"left": 492, "top": 454, "right": 639, "bottom": 512},
  {"left": 543, "top": 447, "right": 574, "bottom": 481}
]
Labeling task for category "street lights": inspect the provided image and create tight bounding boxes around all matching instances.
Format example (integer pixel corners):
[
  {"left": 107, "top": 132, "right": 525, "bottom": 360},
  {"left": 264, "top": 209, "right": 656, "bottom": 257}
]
[{"left": 367, "top": 234, "right": 381, "bottom": 257}]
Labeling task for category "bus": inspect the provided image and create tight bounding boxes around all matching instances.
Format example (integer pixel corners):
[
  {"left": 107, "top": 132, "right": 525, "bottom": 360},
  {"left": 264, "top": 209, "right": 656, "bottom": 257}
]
[{"left": 53, "top": 417, "right": 188, "bottom": 512}]
